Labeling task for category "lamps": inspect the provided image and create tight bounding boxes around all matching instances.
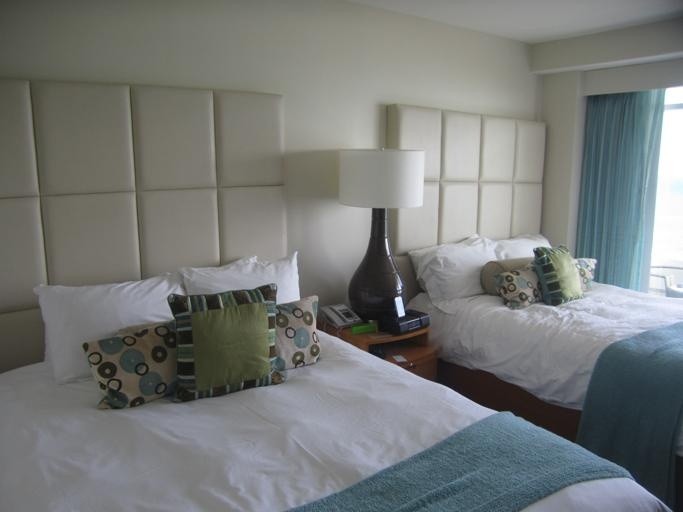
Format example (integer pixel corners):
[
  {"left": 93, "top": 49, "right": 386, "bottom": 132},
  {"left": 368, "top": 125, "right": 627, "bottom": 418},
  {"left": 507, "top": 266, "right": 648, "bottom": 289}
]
[{"left": 339, "top": 149, "right": 425, "bottom": 322}]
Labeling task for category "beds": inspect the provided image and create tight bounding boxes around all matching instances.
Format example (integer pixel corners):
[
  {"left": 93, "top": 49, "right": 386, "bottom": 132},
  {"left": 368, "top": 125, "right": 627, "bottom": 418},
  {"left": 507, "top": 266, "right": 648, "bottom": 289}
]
[
  {"left": 387, "top": 101, "right": 683, "bottom": 458},
  {"left": 0, "top": 78, "right": 672, "bottom": 512}
]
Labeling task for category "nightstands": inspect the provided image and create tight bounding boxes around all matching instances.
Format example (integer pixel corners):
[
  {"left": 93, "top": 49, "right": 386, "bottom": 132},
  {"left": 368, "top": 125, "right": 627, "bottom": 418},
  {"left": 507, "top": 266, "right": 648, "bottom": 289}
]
[{"left": 316, "top": 319, "right": 442, "bottom": 380}]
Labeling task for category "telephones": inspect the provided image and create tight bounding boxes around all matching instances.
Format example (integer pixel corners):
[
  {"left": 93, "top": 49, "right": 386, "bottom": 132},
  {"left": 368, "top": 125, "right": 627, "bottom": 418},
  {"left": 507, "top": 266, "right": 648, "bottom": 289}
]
[{"left": 320, "top": 303, "right": 362, "bottom": 329}]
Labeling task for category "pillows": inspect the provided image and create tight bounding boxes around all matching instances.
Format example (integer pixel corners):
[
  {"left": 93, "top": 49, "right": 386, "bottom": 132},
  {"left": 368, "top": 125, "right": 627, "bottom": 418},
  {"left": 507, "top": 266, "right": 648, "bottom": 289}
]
[{"left": 409, "top": 232, "right": 597, "bottom": 310}]
